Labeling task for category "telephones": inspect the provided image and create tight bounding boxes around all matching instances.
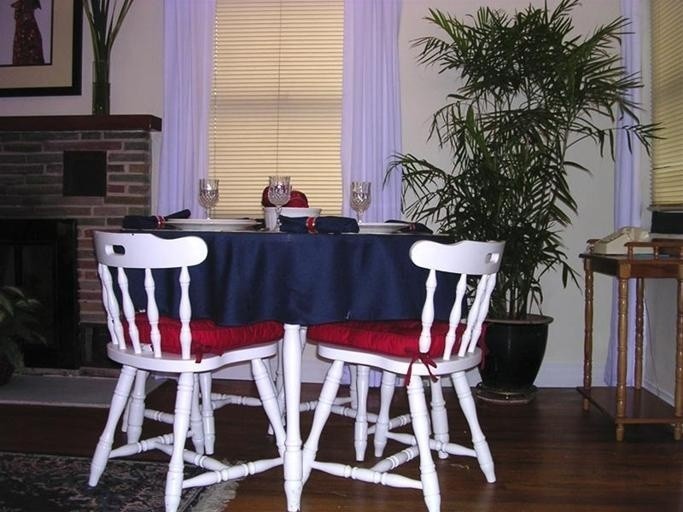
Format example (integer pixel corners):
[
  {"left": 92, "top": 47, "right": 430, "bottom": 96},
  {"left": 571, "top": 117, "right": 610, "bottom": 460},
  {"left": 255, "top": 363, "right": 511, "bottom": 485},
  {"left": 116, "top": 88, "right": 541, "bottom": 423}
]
[{"left": 594, "top": 226, "right": 654, "bottom": 255}]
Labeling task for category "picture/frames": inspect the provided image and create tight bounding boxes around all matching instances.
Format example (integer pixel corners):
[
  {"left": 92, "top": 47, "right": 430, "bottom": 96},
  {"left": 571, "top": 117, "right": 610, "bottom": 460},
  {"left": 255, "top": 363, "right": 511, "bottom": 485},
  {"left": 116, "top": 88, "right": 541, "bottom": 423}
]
[{"left": 0, "top": 1, "right": 84, "bottom": 98}]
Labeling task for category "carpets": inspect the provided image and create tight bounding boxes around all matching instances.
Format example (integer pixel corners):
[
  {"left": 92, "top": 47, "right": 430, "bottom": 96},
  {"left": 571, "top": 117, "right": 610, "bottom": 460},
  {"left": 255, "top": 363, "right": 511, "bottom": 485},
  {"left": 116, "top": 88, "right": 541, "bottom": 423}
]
[{"left": 0, "top": 451, "right": 250, "bottom": 512}]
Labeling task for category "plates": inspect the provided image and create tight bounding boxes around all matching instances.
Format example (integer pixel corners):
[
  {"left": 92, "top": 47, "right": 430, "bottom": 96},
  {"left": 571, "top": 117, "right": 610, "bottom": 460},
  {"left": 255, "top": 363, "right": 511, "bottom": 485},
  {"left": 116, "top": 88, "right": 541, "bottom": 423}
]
[
  {"left": 343, "top": 222, "right": 411, "bottom": 234},
  {"left": 160, "top": 217, "right": 263, "bottom": 231}
]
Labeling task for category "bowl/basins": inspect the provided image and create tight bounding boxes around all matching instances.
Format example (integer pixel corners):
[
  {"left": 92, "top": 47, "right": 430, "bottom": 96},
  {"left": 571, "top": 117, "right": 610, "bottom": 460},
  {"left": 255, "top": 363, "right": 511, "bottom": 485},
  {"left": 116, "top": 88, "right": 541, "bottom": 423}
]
[{"left": 262, "top": 207, "right": 322, "bottom": 232}]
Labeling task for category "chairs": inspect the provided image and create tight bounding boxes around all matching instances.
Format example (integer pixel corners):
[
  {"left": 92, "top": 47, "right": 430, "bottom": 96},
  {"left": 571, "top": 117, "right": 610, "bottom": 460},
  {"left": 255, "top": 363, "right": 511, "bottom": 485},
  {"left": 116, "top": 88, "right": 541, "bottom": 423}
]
[
  {"left": 88, "top": 231, "right": 285, "bottom": 510},
  {"left": 302, "top": 237, "right": 507, "bottom": 510}
]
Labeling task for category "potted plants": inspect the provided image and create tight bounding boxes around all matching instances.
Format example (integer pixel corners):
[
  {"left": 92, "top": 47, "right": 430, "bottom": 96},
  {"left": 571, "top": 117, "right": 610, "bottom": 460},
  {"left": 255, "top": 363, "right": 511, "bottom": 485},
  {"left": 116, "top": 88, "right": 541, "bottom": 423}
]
[
  {"left": 382, "top": 0, "right": 666, "bottom": 403},
  {"left": 0, "top": 286, "right": 48, "bottom": 386},
  {"left": 83, "top": 0, "right": 134, "bottom": 116}
]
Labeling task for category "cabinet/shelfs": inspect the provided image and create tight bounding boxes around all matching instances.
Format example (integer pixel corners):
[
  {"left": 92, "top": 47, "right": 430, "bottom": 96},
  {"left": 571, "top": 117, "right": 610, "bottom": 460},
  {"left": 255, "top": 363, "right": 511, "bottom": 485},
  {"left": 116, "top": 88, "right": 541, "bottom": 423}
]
[{"left": 575, "top": 235, "right": 683, "bottom": 439}]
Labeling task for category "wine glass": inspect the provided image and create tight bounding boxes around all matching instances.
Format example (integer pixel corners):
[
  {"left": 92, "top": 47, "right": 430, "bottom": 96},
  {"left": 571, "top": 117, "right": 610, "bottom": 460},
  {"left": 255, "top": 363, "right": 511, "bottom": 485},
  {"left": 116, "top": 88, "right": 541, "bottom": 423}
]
[
  {"left": 198, "top": 177, "right": 220, "bottom": 220},
  {"left": 267, "top": 175, "right": 292, "bottom": 234},
  {"left": 349, "top": 180, "right": 373, "bottom": 224}
]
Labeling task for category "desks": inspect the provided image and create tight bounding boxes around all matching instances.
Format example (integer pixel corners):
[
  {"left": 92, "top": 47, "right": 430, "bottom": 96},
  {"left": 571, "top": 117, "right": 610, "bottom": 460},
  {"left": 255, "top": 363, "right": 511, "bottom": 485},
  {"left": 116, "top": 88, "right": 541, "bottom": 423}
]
[{"left": 115, "top": 221, "right": 457, "bottom": 511}]
